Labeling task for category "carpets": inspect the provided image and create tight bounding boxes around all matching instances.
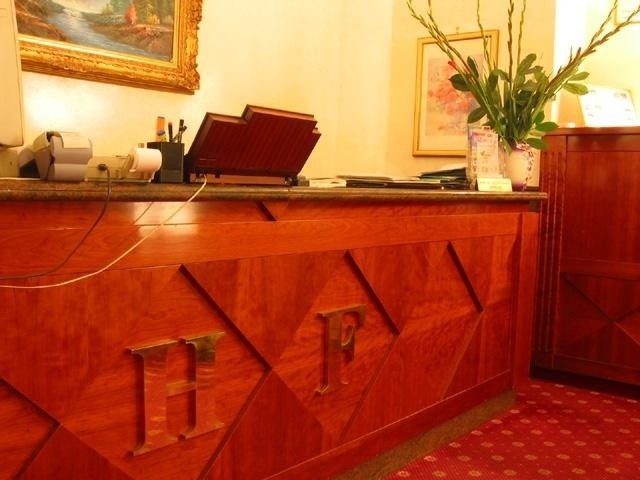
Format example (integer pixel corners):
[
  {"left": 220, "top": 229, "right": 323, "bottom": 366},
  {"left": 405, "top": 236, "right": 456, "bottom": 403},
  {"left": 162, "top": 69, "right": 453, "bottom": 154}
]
[{"left": 382, "top": 380, "right": 640, "bottom": 479}]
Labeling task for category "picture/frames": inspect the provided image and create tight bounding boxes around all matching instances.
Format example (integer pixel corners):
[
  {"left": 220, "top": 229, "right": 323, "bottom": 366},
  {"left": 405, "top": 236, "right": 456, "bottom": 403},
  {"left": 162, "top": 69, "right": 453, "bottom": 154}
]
[
  {"left": 411, "top": 30, "right": 500, "bottom": 158},
  {"left": 15, "top": 0, "right": 202, "bottom": 94}
]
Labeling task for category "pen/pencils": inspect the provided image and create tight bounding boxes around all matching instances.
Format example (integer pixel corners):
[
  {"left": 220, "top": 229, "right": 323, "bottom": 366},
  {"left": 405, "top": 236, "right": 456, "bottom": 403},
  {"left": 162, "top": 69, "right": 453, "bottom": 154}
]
[{"left": 158, "top": 119, "right": 187, "bottom": 144}]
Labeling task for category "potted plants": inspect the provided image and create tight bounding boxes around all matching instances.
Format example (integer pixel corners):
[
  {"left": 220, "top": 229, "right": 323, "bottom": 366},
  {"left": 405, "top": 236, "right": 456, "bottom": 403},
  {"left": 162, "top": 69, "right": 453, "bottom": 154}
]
[{"left": 407, "top": 0, "right": 640, "bottom": 193}]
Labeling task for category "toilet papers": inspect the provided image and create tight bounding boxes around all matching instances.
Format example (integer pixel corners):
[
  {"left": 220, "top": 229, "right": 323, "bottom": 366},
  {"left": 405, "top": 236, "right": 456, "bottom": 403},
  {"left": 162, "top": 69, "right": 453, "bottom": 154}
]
[{"left": 128, "top": 147, "right": 162, "bottom": 172}]
